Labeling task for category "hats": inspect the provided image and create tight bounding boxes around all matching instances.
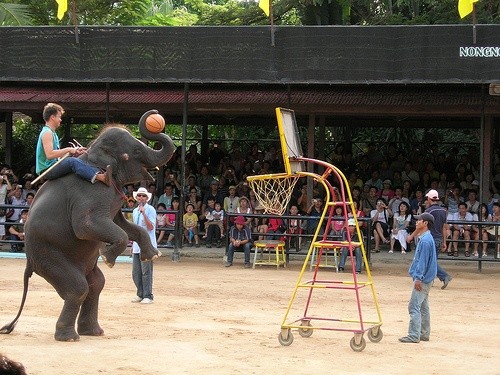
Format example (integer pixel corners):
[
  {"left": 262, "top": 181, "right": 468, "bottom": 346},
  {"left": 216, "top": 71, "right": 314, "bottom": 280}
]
[
  {"left": 229, "top": 186, "right": 236, "bottom": 190},
  {"left": 382, "top": 180, "right": 391, "bottom": 184},
  {"left": 348, "top": 219, "right": 356, "bottom": 225},
  {"left": 413, "top": 213, "right": 435, "bottom": 223},
  {"left": 472, "top": 181, "right": 479, "bottom": 185},
  {"left": 132, "top": 188, "right": 152, "bottom": 202},
  {"left": 425, "top": 190, "right": 439, "bottom": 200},
  {"left": 234, "top": 216, "right": 245, "bottom": 224}
]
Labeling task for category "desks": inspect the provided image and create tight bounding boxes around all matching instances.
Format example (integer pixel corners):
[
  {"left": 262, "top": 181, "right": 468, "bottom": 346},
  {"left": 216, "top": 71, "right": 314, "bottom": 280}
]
[
  {"left": 310, "top": 240, "right": 341, "bottom": 272},
  {"left": 253, "top": 240, "right": 286, "bottom": 270}
]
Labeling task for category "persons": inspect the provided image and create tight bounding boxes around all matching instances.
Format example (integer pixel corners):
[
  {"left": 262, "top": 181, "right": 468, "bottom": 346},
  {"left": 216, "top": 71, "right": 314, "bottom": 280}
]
[
  {"left": 132, "top": 188, "right": 157, "bottom": 304},
  {"left": 36, "top": 103, "right": 113, "bottom": 186},
  {"left": 398, "top": 213, "right": 438, "bottom": 343},
  {"left": 224, "top": 215, "right": 253, "bottom": 268},
  {"left": 0, "top": 163, "right": 44, "bottom": 253},
  {"left": 339, "top": 219, "right": 362, "bottom": 274},
  {"left": 406, "top": 189, "right": 452, "bottom": 289},
  {"left": 123, "top": 141, "right": 500, "bottom": 254}
]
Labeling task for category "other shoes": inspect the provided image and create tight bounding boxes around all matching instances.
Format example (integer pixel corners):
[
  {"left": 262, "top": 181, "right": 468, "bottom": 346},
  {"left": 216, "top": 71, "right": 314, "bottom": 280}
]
[
  {"left": 371, "top": 249, "right": 380, "bottom": 253},
  {"left": 398, "top": 336, "right": 428, "bottom": 343},
  {"left": 131, "top": 296, "right": 152, "bottom": 304},
  {"left": 454, "top": 251, "right": 470, "bottom": 256},
  {"left": 383, "top": 239, "right": 390, "bottom": 244},
  {"left": 389, "top": 250, "right": 393, "bottom": 253},
  {"left": 10, "top": 247, "right": 17, "bottom": 252},
  {"left": 167, "top": 240, "right": 172, "bottom": 246},
  {"left": 225, "top": 262, "right": 250, "bottom": 268},
  {"left": 401, "top": 251, "right": 406, "bottom": 254},
  {"left": 473, "top": 251, "right": 487, "bottom": 257},
  {"left": 448, "top": 252, "right": 454, "bottom": 256},
  {"left": 206, "top": 242, "right": 221, "bottom": 248},
  {"left": 188, "top": 243, "right": 200, "bottom": 248},
  {"left": 441, "top": 275, "right": 452, "bottom": 289},
  {"left": 104, "top": 165, "right": 112, "bottom": 185}
]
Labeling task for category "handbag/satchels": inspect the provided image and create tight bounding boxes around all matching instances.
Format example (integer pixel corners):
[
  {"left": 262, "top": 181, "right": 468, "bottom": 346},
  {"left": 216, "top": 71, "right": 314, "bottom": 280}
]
[{"left": 6, "top": 209, "right": 14, "bottom": 217}]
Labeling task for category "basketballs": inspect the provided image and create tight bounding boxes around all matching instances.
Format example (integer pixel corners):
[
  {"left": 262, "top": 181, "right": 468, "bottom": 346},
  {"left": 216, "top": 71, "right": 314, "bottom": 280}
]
[{"left": 145, "top": 114, "right": 165, "bottom": 133}]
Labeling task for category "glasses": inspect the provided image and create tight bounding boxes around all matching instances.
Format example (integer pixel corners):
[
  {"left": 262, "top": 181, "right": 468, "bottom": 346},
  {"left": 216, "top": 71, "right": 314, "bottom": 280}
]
[
  {"left": 230, "top": 202, "right": 232, "bottom": 208},
  {"left": 138, "top": 194, "right": 145, "bottom": 197}
]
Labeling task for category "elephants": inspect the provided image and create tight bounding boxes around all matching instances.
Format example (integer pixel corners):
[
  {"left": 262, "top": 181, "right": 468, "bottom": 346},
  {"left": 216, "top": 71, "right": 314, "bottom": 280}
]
[{"left": 0, "top": 108, "right": 176, "bottom": 344}]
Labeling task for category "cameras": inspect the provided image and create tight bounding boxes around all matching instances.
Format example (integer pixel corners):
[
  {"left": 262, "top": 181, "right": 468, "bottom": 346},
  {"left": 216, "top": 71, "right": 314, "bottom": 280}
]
[
  {"left": 313, "top": 199, "right": 317, "bottom": 203},
  {"left": 169, "top": 175, "right": 174, "bottom": 178},
  {"left": 18, "top": 185, "right": 22, "bottom": 188},
  {"left": 5, "top": 170, "right": 10, "bottom": 173},
  {"left": 377, "top": 202, "right": 381, "bottom": 205},
  {"left": 0, "top": 176, "right": 3, "bottom": 179}
]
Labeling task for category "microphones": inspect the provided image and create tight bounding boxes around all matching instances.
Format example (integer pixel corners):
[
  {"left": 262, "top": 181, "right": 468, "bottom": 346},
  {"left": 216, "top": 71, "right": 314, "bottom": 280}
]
[{"left": 139, "top": 202, "right": 144, "bottom": 213}]
